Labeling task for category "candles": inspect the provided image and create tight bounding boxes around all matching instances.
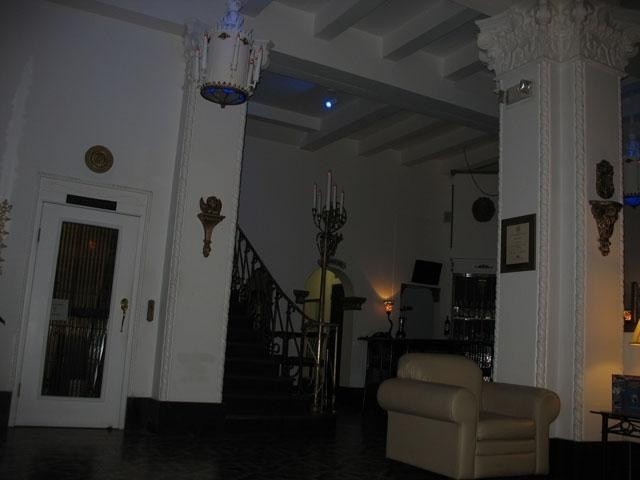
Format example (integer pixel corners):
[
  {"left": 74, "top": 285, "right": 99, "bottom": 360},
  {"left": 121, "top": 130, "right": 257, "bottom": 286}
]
[{"left": 312, "top": 169, "right": 346, "bottom": 215}]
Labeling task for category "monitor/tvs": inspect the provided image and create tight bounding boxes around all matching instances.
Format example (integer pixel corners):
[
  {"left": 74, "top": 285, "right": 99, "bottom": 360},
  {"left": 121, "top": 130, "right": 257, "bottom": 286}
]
[{"left": 452, "top": 272, "right": 496, "bottom": 320}]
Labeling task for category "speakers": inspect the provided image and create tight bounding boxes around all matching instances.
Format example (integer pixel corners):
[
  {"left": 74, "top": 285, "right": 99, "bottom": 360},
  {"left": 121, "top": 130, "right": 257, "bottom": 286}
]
[{"left": 411, "top": 260, "right": 443, "bottom": 285}]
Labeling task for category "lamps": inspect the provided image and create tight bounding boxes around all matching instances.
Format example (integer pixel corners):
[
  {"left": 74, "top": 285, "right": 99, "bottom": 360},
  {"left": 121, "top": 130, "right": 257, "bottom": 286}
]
[
  {"left": 372, "top": 300, "right": 393, "bottom": 336},
  {"left": 196, "top": 1, "right": 264, "bottom": 107},
  {"left": 629, "top": 319, "right": 640, "bottom": 346}
]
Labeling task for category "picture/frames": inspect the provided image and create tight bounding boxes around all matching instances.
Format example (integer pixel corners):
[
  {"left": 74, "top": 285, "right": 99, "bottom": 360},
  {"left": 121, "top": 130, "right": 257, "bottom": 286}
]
[{"left": 501, "top": 213, "right": 535, "bottom": 272}]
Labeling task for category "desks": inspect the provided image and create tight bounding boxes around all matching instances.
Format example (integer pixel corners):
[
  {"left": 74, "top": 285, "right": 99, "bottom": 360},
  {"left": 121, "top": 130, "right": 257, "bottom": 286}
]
[
  {"left": 357, "top": 337, "right": 471, "bottom": 418},
  {"left": 589, "top": 409, "right": 640, "bottom": 458}
]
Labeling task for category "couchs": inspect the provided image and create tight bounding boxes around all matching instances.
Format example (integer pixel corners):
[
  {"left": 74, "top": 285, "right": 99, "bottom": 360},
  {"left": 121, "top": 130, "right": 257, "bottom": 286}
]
[{"left": 376, "top": 353, "right": 560, "bottom": 480}]
